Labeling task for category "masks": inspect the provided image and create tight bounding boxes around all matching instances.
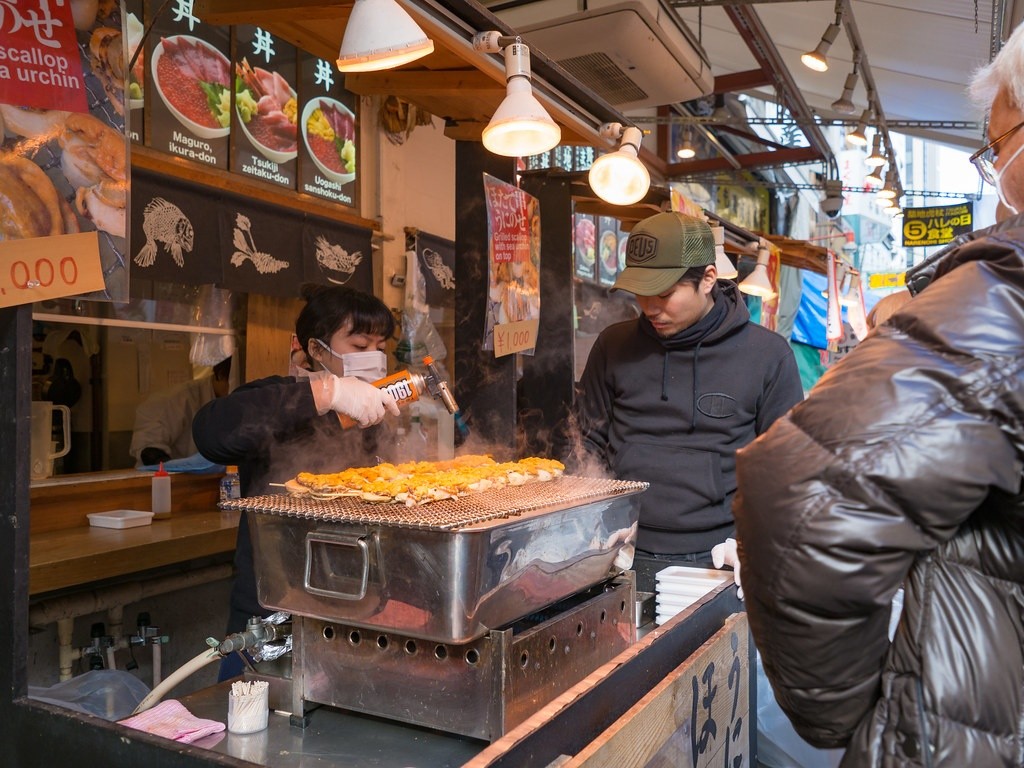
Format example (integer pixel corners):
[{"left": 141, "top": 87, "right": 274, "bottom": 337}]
[
  {"left": 313, "top": 339, "right": 387, "bottom": 384},
  {"left": 994, "top": 144, "right": 1023, "bottom": 214}
]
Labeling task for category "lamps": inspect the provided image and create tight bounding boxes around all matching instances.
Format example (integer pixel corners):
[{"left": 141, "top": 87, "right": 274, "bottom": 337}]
[
  {"left": 821, "top": 259, "right": 845, "bottom": 300},
  {"left": 831, "top": 51, "right": 862, "bottom": 114},
  {"left": 589, "top": 122, "right": 652, "bottom": 205},
  {"left": 736, "top": 242, "right": 774, "bottom": 298},
  {"left": 472, "top": 30, "right": 560, "bottom": 157},
  {"left": 677, "top": 121, "right": 695, "bottom": 159},
  {"left": 842, "top": 274, "right": 858, "bottom": 307},
  {"left": 802, "top": 11, "right": 843, "bottom": 74},
  {"left": 705, "top": 220, "right": 737, "bottom": 280},
  {"left": 863, "top": 121, "right": 886, "bottom": 168},
  {"left": 879, "top": 168, "right": 902, "bottom": 217},
  {"left": 845, "top": 89, "right": 875, "bottom": 146},
  {"left": 866, "top": 150, "right": 889, "bottom": 187},
  {"left": 337, "top": 0, "right": 435, "bottom": 72}
]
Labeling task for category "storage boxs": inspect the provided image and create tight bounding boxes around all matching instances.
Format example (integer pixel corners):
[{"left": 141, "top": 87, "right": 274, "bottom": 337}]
[
  {"left": 653, "top": 566, "right": 734, "bottom": 626},
  {"left": 86, "top": 509, "right": 154, "bottom": 530}
]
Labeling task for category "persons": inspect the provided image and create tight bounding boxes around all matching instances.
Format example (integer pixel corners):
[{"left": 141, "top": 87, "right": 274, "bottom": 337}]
[
  {"left": 559, "top": 211, "right": 805, "bottom": 602},
  {"left": 736, "top": 20, "right": 1024, "bottom": 768},
  {"left": 191, "top": 283, "right": 400, "bottom": 686}
]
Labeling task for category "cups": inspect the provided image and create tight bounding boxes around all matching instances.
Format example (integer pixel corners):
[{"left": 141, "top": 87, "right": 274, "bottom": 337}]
[
  {"left": 49, "top": 441, "right": 60, "bottom": 476},
  {"left": 228, "top": 686, "right": 271, "bottom": 733}
]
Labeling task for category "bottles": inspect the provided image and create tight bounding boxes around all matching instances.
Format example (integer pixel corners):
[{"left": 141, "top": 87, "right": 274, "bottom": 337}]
[
  {"left": 219, "top": 466, "right": 242, "bottom": 511},
  {"left": 336, "top": 369, "right": 432, "bottom": 431},
  {"left": 151, "top": 461, "right": 172, "bottom": 520}
]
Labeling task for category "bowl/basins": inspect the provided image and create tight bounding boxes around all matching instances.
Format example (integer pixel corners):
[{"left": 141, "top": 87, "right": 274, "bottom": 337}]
[
  {"left": 301, "top": 96, "right": 357, "bottom": 185},
  {"left": 599, "top": 230, "right": 618, "bottom": 275},
  {"left": 151, "top": 34, "right": 231, "bottom": 139},
  {"left": 235, "top": 88, "right": 298, "bottom": 164},
  {"left": 618, "top": 237, "right": 629, "bottom": 271},
  {"left": 87, "top": 509, "right": 155, "bottom": 529},
  {"left": 577, "top": 218, "right": 595, "bottom": 266},
  {"left": 654, "top": 566, "right": 735, "bottom": 626}
]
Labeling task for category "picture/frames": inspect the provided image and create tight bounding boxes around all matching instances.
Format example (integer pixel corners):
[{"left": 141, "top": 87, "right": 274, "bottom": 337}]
[{"left": 657, "top": 104, "right": 779, "bottom": 236}]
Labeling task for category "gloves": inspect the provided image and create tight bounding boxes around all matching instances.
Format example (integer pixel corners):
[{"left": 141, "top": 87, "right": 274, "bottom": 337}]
[
  {"left": 712, "top": 537, "right": 746, "bottom": 602},
  {"left": 328, "top": 372, "right": 401, "bottom": 429}
]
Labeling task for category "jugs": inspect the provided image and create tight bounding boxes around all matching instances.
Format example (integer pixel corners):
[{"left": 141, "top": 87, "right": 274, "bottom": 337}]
[{"left": 30, "top": 401, "right": 70, "bottom": 480}]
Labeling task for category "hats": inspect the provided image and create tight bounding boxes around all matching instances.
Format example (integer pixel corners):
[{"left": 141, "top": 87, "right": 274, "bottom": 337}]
[{"left": 610, "top": 209, "right": 716, "bottom": 296}]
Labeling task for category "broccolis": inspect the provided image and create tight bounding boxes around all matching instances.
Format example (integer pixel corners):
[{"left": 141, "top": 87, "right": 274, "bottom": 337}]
[
  {"left": 341, "top": 139, "right": 358, "bottom": 172},
  {"left": 215, "top": 88, "right": 259, "bottom": 127}
]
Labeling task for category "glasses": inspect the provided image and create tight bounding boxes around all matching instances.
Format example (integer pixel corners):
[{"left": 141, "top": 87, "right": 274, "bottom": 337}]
[{"left": 969, "top": 119, "right": 1024, "bottom": 186}]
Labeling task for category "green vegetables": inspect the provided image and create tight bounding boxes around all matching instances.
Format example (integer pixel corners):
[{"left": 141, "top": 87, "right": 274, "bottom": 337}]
[
  {"left": 199, "top": 76, "right": 257, "bottom": 118},
  {"left": 335, "top": 136, "right": 356, "bottom": 163}
]
